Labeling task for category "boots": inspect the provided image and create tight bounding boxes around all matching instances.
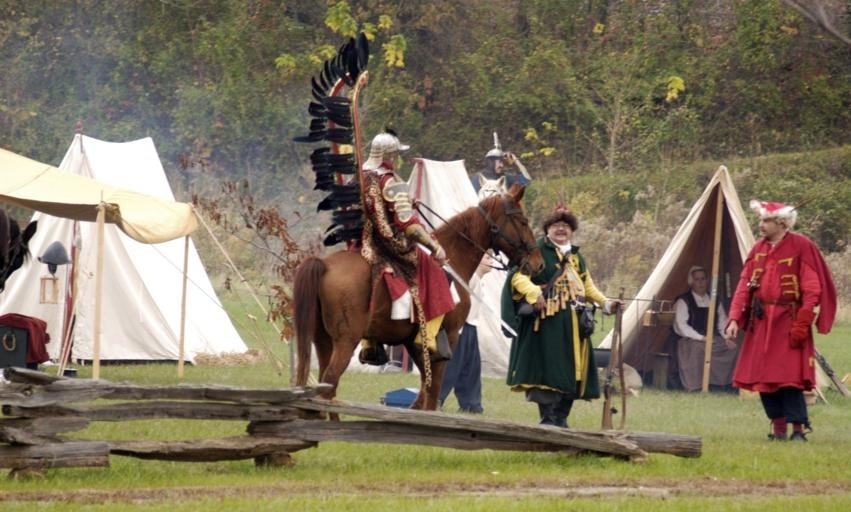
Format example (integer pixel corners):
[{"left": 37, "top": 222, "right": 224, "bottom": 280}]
[
  {"left": 412, "top": 303, "right": 454, "bottom": 362},
  {"left": 356, "top": 339, "right": 391, "bottom": 367}
]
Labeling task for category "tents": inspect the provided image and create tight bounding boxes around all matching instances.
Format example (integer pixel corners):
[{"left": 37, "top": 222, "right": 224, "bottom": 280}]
[
  {"left": 306, "top": 155, "right": 514, "bottom": 379},
  {"left": 586, "top": 163, "right": 827, "bottom": 404},
  {"left": 0, "top": 133, "right": 249, "bottom": 364}
]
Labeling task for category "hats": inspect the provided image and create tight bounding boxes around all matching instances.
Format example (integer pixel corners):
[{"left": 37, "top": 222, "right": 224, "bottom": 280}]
[
  {"left": 540, "top": 207, "right": 579, "bottom": 233},
  {"left": 747, "top": 197, "right": 801, "bottom": 230}
]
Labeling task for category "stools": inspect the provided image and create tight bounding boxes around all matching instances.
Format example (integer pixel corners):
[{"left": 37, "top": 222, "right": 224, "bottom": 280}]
[{"left": 652, "top": 351, "right": 671, "bottom": 391}]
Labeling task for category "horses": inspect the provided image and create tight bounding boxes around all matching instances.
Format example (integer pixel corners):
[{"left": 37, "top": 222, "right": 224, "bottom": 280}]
[
  {"left": 289, "top": 180, "right": 546, "bottom": 424},
  {"left": 1, "top": 206, "right": 39, "bottom": 294}
]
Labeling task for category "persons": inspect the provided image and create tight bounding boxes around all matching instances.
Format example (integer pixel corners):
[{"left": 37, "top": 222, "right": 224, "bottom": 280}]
[
  {"left": 671, "top": 264, "right": 746, "bottom": 394},
  {"left": 434, "top": 249, "right": 495, "bottom": 413},
  {"left": 500, "top": 200, "right": 628, "bottom": 429},
  {"left": 343, "top": 132, "right": 463, "bottom": 366},
  {"left": 469, "top": 130, "right": 533, "bottom": 207},
  {"left": 726, "top": 196, "right": 840, "bottom": 442}
]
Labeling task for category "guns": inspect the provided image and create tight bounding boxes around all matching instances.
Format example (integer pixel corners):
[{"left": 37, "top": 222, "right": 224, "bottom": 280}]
[{"left": 601, "top": 288, "right": 625, "bottom": 430}]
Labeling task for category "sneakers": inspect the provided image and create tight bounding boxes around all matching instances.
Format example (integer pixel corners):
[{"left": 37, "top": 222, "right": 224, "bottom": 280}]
[
  {"left": 789, "top": 431, "right": 809, "bottom": 443},
  {"left": 768, "top": 432, "right": 787, "bottom": 443}
]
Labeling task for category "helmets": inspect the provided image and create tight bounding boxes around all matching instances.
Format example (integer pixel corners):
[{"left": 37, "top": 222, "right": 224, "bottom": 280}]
[
  {"left": 361, "top": 130, "right": 411, "bottom": 172},
  {"left": 484, "top": 130, "right": 509, "bottom": 177}
]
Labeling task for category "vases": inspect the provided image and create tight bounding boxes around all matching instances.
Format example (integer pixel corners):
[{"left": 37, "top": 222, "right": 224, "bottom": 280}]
[{"left": 593, "top": 348, "right": 612, "bottom": 367}]
